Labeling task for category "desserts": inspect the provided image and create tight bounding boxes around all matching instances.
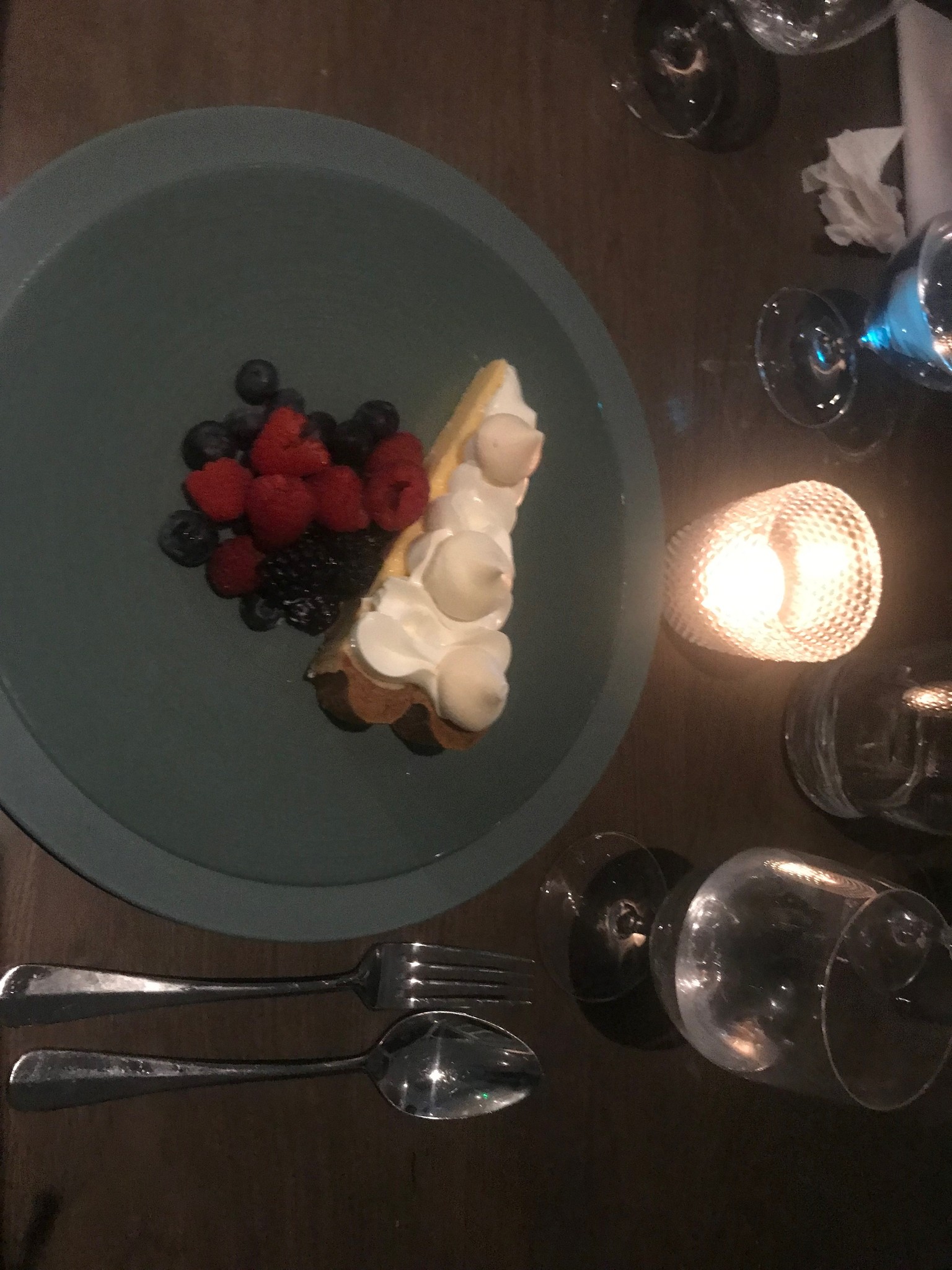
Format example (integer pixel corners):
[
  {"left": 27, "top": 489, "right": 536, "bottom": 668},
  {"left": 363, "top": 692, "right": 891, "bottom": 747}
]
[{"left": 304, "top": 359, "right": 545, "bottom": 752}]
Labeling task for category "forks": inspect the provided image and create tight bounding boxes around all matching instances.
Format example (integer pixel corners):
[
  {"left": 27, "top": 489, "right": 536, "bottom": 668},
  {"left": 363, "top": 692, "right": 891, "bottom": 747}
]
[{"left": 1, "top": 942, "right": 535, "bottom": 1029}]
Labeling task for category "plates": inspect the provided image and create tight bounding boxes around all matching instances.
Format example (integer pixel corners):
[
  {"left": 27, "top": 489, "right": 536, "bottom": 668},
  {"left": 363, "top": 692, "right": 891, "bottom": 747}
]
[{"left": 0, "top": 105, "right": 667, "bottom": 942}]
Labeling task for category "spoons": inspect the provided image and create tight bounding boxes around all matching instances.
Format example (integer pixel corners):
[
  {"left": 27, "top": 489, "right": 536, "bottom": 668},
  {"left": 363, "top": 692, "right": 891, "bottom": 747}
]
[{"left": 8, "top": 1011, "right": 542, "bottom": 1119}]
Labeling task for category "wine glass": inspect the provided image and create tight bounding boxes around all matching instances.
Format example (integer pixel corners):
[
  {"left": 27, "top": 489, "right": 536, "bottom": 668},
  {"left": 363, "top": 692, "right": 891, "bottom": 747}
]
[
  {"left": 756, "top": 211, "right": 952, "bottom": 428},
  {"left": 534, "top": 831, "right": 952, "bottom": 1113},
  {"left": 595, "top": 0, "right": 911, "bottom": 140}
]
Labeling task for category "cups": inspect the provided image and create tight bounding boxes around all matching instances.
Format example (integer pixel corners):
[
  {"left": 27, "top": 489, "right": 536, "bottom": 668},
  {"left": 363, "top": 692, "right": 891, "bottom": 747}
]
[{"left": 786, "top": 637, "right": 952, "bottom": 832}]
